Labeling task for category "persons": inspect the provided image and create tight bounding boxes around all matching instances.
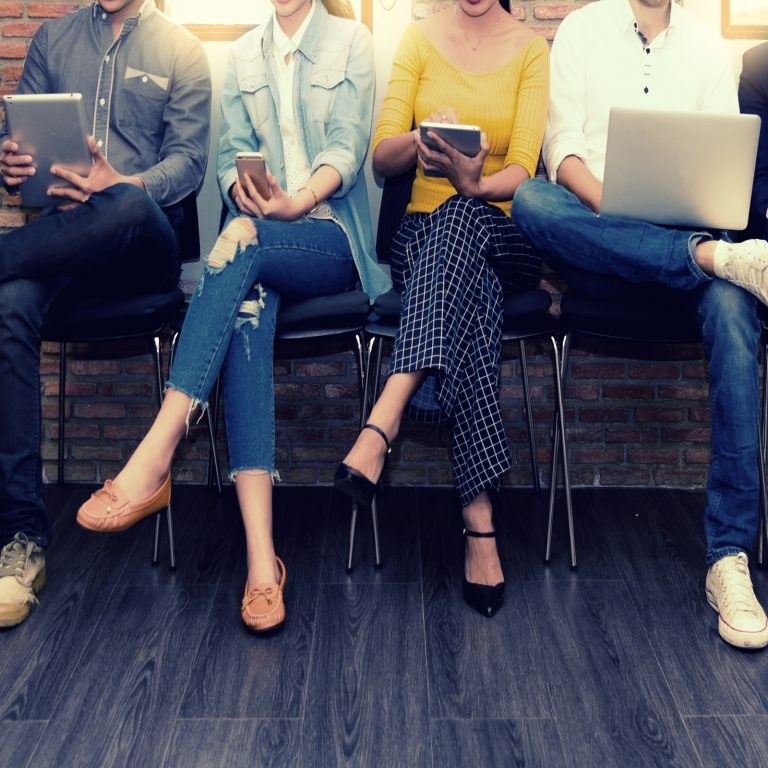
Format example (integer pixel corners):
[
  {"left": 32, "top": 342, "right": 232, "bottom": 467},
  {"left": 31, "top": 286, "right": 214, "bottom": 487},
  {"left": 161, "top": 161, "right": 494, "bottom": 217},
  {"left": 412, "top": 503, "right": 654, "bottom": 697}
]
[
  {"left": 76, "top": 0, "right": 394, "bottom": 631},
  {"left": 510, "top": 0, "right": 768, "bottom": 652},
  {"left": 0, "top": 0, "right": 212, "bottom": 627},
  {"left": 333, "top": 0, "right": 551, "bottom": 617}
]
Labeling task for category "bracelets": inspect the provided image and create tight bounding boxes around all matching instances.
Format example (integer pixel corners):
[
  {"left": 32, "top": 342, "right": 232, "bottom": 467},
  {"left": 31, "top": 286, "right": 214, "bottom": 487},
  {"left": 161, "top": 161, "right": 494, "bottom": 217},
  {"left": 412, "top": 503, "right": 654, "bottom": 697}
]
[{"left": 298, "top": 187, "right": 320, "bottom": 218}]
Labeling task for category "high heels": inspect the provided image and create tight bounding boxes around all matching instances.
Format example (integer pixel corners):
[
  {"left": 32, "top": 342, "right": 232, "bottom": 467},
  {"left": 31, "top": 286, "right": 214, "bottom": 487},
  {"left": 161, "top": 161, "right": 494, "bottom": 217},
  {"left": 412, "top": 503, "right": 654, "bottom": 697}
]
[
  {"left": 334, "top": 424, "right": 391, "bottom": 508},
  {"left": 462, "top": 529, "right": 506, "bottom": 616}
]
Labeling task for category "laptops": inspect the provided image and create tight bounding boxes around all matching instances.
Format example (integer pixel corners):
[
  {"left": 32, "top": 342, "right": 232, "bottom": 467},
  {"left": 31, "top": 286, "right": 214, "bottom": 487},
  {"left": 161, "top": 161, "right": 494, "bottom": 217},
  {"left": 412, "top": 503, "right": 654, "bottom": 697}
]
[{"left": 599, "top": 105, "right": 762, "bottom": 230}]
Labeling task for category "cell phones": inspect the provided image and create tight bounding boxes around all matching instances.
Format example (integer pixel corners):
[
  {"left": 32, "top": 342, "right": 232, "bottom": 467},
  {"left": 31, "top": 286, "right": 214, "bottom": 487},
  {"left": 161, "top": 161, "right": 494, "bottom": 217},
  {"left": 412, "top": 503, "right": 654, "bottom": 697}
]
[{"left": 235, "top": 152, "right": 271, "bottom": 202}]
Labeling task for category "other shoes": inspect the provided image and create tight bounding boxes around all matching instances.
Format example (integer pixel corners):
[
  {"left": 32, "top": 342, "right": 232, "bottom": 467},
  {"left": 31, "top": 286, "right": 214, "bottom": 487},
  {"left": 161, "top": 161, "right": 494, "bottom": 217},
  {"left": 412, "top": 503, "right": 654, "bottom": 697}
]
[
  {"left": 242, "top": 555, "right": 286, "bottom": 631},
  {"left": 76, "top": 471, "right": 172, "bottom": 533}
]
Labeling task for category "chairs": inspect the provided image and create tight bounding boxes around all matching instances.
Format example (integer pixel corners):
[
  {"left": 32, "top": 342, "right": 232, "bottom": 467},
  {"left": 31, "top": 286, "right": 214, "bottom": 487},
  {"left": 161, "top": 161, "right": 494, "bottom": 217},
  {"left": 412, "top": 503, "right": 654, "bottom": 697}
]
[{"left": 57, "top": 265, "right": 768, "bottom": 573}]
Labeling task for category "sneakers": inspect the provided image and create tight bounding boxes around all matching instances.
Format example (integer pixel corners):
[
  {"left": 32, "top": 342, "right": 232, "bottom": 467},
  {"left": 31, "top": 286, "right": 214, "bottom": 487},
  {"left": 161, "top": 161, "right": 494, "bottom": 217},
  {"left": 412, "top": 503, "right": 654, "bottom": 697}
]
[
  {"left": 0, "top": 532, "right": 46, "bottom": 628},
  {"left": 713, "top": 238, "right": 768, "bottom": 306},
  {"left": 705, "top": 552, "right": 768, "bottom": 649}
]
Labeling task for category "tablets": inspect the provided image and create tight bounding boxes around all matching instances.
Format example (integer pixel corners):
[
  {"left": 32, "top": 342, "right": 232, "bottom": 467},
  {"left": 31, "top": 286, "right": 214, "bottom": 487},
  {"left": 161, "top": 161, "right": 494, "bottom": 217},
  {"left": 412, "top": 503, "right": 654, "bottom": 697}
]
[
  {"left": 3, "top": 92, "right": 92, "bottom": 209},
  {"left": 420, "top": 122, "right": 480, "bottom": 176}
]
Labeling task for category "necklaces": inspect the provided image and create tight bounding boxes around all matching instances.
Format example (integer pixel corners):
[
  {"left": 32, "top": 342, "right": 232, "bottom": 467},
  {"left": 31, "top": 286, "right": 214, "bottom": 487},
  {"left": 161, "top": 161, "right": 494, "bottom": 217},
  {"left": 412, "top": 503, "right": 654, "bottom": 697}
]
[{"left": 453, "top": 3, "right": 503, "bottom": 51}]
